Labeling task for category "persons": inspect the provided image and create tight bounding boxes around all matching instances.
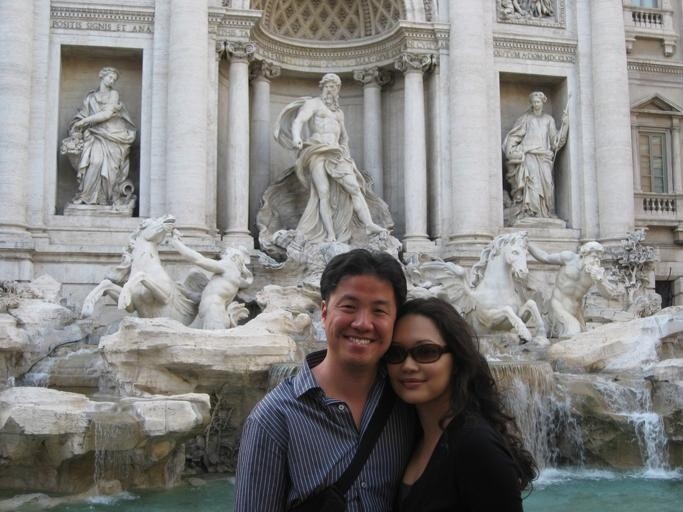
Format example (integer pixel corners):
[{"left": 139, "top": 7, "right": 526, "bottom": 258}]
[
  {"left": 235, "top": 250, "right": 418, "bottom": 512},
  {"left": 170, "top": 231, "right": 251, "bottom": 329},
  {"left": 290, "top": 74, "right": 392, "bottom": 244},
  {"left": 385, "top": 297, "right": 538, "bottom": 511},
  {"left": 523, "top": 236, "right": 617, "bottom": 340},
  {"left": 499, "top": 91, "right": 575, "bottom": 221},
  {"left": 61, "top": 64, "right": 134, "bottom": 203}
]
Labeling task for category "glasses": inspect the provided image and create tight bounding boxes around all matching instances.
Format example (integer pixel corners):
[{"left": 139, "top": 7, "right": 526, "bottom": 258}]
[{"left": 387, "top": 343, "right": 455, "bottom": 365}]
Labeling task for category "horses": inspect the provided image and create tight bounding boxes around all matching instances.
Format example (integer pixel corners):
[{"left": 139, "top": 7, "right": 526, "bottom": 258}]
[
  {"left": 463, "top": 230, "right": 548, "bottom": 344},
  {"left": 79, "top": 212, "right": 249, "bottom": 329}
]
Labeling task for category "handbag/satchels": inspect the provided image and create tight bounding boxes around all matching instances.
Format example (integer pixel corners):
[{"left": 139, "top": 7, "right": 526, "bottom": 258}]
[{"left": 291, "top": 486, "right": 345, "bottom": 512}]
